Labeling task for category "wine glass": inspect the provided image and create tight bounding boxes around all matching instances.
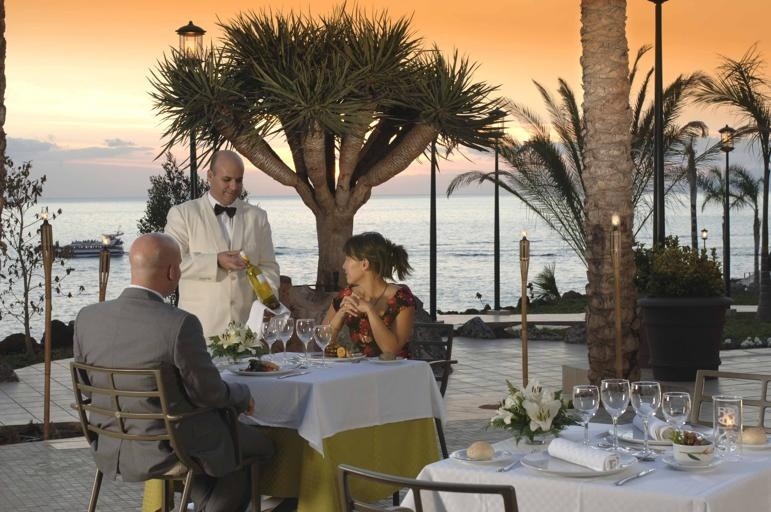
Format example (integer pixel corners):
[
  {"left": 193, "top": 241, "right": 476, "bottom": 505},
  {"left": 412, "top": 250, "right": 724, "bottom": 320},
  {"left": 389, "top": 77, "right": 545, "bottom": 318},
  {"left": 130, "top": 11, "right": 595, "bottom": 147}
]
[
  {"left": 572, "top": 384, "right": 600, "bottom": 447},
  {"left": 599, "top": 377, "right": 632, "bottom": 455},
  {"left": 261, "top": 317, "right": 278, "bottom": 361},
  {"left": 630, "top": 379, "right": 660, "bottom": 460},
  {"left": 312, "top": 324, "right": 333, "bottom": 367},
  {"left": 295, "top": 318, "right": 316, "bottom": 362},
  {"left": 275, "top": 319, "right": 295, "bottom": 360}
]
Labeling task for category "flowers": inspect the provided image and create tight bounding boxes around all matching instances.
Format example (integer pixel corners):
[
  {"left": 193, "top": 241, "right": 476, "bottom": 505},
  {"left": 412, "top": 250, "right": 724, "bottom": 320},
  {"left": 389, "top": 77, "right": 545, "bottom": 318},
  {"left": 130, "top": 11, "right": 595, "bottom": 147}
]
[
  {"left": 207, "top": 320, "right": 264, "bottom": 363},
  {"left": 482, "top": 379, "right": 584, "bottom": 445}
]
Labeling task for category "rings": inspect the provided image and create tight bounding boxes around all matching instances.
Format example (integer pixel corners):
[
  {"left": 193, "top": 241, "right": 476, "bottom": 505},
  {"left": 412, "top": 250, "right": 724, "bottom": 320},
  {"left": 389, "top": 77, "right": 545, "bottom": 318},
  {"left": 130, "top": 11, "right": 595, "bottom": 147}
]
[
  {"left": 230, "top": 269, "right": 233, "bottom": 274},
  {"left": 345, "top": 302, "right": 348, "bottom": 307}
]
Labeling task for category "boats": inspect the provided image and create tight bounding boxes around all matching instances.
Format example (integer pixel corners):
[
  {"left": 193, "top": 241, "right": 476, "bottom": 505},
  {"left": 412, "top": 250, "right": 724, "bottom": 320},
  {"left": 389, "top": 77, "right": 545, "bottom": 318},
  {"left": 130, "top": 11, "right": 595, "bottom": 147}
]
[{"left": 52, "top": 231, "right": 128, "bottom": 259}]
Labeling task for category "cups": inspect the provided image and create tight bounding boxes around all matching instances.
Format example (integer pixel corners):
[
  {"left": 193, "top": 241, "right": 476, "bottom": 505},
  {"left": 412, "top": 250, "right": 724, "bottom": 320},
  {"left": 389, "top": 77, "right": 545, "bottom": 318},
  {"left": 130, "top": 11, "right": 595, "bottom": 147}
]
[
  {"left": 661, "top": 390, "right": 693, "bottom": 429},
  {"left": 710, "top": 392, "right": 744, "bottom": 464}
]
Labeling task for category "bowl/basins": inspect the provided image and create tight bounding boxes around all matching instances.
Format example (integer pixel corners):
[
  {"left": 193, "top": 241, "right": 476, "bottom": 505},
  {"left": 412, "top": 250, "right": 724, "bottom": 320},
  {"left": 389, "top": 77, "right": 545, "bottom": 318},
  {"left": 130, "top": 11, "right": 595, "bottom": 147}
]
[{"left": 672, "top": 440, "right": 715, "bottom": 466}]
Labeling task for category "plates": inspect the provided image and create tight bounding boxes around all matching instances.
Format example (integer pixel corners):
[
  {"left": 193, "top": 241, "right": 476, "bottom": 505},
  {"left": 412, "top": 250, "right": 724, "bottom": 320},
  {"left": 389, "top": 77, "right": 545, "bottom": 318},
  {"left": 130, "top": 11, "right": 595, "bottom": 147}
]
[
  {"left": 662, "top": 451, "right": 722, "bottom": 470},
  {"left": 609, "top": 420, "right": 690, "bottom": 446},
  {"left": 226, "top": 359, "right": 298, "bottom": 377},
  {"left": 449, "top": 446, "right": 510, "bottom": 467},
  {"left": 368, "top": 355, "right": 409, "bottom": 364},
  {"left": 522, "top": 447, "right": 637, "bottom": 478},
  {"left": 307, "top": 352, "right": 367, "bottom": 362},
  {"left": 736, "top": 433, "right": 770, "bottom": 450}
]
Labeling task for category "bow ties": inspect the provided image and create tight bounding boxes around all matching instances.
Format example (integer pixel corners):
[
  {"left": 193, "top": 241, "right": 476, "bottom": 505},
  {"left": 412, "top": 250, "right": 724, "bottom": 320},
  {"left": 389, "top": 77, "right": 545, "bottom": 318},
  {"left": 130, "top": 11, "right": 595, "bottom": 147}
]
[{"left": 214, "top": 204, "right": 237, "bottom": 218}]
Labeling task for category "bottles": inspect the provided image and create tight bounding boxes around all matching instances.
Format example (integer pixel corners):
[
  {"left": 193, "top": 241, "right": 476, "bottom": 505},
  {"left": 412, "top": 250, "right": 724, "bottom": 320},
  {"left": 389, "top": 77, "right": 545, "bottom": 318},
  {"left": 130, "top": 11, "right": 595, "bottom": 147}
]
[{"left": 238, "top": 248, "right": 280, "bottom": 310}]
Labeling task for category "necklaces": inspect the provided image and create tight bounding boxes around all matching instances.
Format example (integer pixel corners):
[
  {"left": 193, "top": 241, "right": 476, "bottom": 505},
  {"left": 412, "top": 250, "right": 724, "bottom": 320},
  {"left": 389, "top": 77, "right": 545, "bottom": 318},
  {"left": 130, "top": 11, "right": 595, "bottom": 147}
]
[{"left": 356, "top": 285, "right": 388, "bottom": 318}]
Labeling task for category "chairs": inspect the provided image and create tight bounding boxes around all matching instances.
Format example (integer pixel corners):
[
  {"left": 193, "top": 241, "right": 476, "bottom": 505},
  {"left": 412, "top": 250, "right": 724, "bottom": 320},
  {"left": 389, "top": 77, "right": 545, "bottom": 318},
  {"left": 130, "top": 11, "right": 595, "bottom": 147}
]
[
  {"left": 69, "top": 360, "right": 261, "bottom": 512},
  {"left": 689, "top": 370, "right": 771, "bottom": 433},
  {"left": 409, "top": 323, "right": 457, "bottom": 459},
  {"left": 337, "top": 464, "right": 518, "bottom": 512}
]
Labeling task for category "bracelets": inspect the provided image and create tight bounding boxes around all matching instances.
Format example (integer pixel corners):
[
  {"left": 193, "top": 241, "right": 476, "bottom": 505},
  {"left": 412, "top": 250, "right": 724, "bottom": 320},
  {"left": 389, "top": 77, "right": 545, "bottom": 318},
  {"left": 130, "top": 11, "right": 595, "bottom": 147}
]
[{"left": 332, "top": 322, "right": 343, "bottom": 331}]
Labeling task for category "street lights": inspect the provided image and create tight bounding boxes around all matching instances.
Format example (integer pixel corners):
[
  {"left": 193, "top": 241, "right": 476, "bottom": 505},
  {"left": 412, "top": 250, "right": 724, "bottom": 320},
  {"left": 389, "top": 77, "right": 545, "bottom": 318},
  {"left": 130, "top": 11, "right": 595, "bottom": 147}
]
[
  {"left": 715, "top": 123, "right": 741, "bottom": 311},
  {"left": 699, "top": 226, "right": 709, "bottom": 256},
  {"left": 173, "top": 18, "right": 211, "bottom": 202},
  {"left": 486, "top": 108, "right": 508, "bottom": 313}
]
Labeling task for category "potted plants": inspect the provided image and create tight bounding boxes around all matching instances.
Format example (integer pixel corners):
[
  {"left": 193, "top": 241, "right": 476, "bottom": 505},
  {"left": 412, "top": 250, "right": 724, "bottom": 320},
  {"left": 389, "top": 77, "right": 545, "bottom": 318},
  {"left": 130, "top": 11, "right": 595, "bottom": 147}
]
[{"left": 634, "top": 235, "right": 734, "bottom": 382}]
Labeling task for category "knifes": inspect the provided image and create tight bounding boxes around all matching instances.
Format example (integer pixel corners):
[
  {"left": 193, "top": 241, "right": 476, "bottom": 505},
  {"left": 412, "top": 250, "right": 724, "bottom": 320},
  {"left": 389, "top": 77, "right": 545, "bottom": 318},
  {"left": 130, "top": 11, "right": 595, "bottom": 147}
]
[
  {"left": 497, "top": 456, "right": 526, "bottom": 475},
  {"left": 278, "top": 371, "right": 312, "bottom": 378},
  {"left": 615, "top": 467, "right": 656, "bottom": 487}
]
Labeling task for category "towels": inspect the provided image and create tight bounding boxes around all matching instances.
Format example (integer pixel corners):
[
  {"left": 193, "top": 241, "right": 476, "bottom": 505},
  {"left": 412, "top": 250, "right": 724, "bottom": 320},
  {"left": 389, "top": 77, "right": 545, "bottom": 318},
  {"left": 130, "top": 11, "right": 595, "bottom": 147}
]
[
  {"left": 548, "top": 438, "right": 621, "bottom": 472},
  {"left": 631, "top": 414, "right": 677, "bottom": 441},
  {"left": 245, "top": 300, "right": 292, "bottom": 340}
]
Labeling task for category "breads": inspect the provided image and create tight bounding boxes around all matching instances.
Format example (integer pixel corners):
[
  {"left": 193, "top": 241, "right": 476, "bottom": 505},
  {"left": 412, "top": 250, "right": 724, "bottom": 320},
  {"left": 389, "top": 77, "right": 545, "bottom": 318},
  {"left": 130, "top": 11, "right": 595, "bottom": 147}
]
[
  {"left": 467, "top": 441, "right": 494, "bottom": 460},
  {"left": 742, "top": 427, "right": 767, "bottom": 444}
]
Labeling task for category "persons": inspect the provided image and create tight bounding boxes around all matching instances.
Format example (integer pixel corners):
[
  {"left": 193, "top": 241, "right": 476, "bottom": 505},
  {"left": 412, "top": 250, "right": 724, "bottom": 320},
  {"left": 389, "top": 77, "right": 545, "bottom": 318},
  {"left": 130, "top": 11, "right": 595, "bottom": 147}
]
[
  {"left": 165, "top": 150, "right": 282, "bottom": 357},
  {"left": 73, "top": 234, "right": 271, "bottom": 511},
  {"left": 316, "top": 232, "right": 418, "bottom": 360}
]
